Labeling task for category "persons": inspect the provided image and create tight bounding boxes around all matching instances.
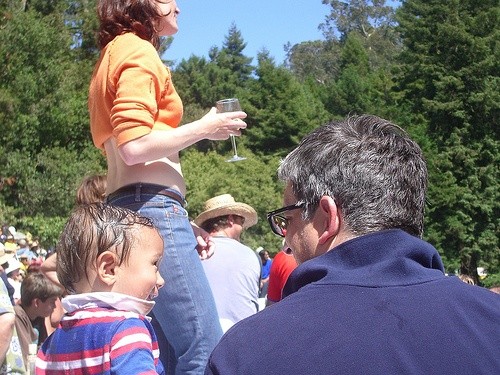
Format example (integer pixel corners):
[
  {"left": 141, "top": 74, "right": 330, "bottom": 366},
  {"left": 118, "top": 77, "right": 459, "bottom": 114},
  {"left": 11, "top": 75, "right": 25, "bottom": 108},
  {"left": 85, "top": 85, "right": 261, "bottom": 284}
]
[
  {"left": 35, "top": 203, "right": 165, "bottom": 375},
  {"left": 89, "top": 0, "right": 247, "bottom": 375},
  {"left": 193, "top": 194, "right": 261, "bottom": 334},
  {"left": 255, "top": 239, "right": 298, "bottom": 312},
  {"left": 205, "top": 117, "right": 500, "bottom": 375},
  {"left": 0, "top": 226, "right": 72, "bottom": 375}
]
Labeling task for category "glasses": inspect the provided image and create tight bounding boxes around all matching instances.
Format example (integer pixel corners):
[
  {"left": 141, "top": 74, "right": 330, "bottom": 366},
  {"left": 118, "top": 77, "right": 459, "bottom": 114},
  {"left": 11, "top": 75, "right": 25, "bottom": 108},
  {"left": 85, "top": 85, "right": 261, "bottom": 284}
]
[{"left": 267, "top": 203, "right": 306, "bottom": 237}]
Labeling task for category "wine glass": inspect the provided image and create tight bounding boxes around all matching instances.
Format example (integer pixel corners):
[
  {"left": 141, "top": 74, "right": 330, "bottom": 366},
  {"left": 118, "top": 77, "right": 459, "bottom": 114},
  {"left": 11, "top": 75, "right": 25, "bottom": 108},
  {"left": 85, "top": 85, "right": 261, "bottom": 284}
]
[{"left": 213, "top": 99, "right": 248, "bottom": 163}]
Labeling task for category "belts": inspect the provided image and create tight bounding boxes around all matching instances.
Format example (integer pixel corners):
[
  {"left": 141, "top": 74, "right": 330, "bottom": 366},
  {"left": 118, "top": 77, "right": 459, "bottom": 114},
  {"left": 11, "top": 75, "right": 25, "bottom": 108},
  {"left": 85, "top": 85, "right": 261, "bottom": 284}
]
[{"left": 107, "top": 188, "right": 185, "bottom": 207}]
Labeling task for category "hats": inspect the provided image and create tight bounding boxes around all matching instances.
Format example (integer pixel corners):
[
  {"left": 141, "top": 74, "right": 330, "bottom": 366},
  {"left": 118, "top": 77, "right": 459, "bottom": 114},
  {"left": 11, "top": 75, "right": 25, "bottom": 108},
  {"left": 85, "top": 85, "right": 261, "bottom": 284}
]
[
  {"left": 194, "top": 194, "right": 258, "bottom": 230},
  {"left": 1, "top": 258, "right": 22, "bottom": 275}
]
